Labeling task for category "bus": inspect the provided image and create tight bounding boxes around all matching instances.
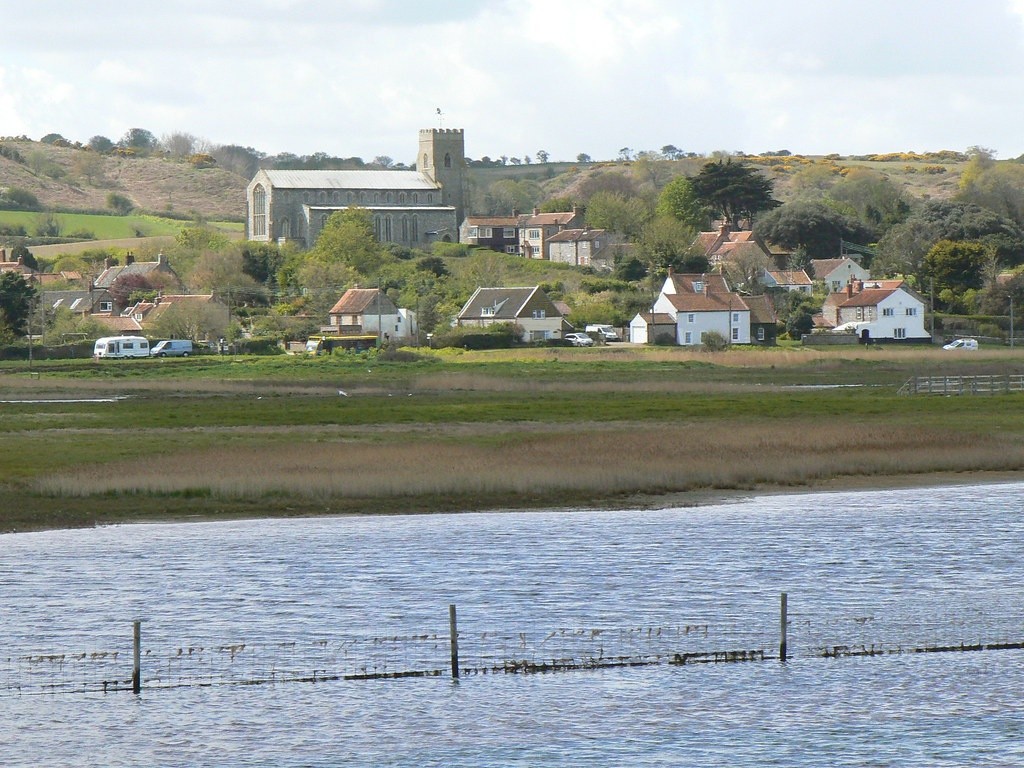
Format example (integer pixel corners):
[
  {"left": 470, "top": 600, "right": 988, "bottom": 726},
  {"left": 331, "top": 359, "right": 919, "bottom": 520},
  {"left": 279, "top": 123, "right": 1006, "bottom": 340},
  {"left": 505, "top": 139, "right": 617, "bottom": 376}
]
[{"left": 94, "top": 336, "right": 150, "bottom": 359}]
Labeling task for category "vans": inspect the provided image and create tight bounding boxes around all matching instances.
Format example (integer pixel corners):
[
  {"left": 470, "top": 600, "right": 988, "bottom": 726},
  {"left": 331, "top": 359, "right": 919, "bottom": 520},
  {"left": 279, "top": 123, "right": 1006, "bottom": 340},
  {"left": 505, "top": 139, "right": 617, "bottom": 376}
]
[
  {"left": 585, "top": 324, "right": 619, "bottom": 341},
  {"left": 150, "top": 339, "right": 193, "bottom": 357}
]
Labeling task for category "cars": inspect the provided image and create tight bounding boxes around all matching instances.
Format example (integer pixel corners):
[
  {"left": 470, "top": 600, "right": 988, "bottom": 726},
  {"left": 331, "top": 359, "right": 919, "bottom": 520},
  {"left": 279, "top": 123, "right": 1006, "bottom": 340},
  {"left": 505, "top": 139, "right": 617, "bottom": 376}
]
[
  {"left": 943, "top": 339, "right": 979, "bottom": 351},
  {"left": 565, "top": 333, "right": 593, "bottom": 347}
]
